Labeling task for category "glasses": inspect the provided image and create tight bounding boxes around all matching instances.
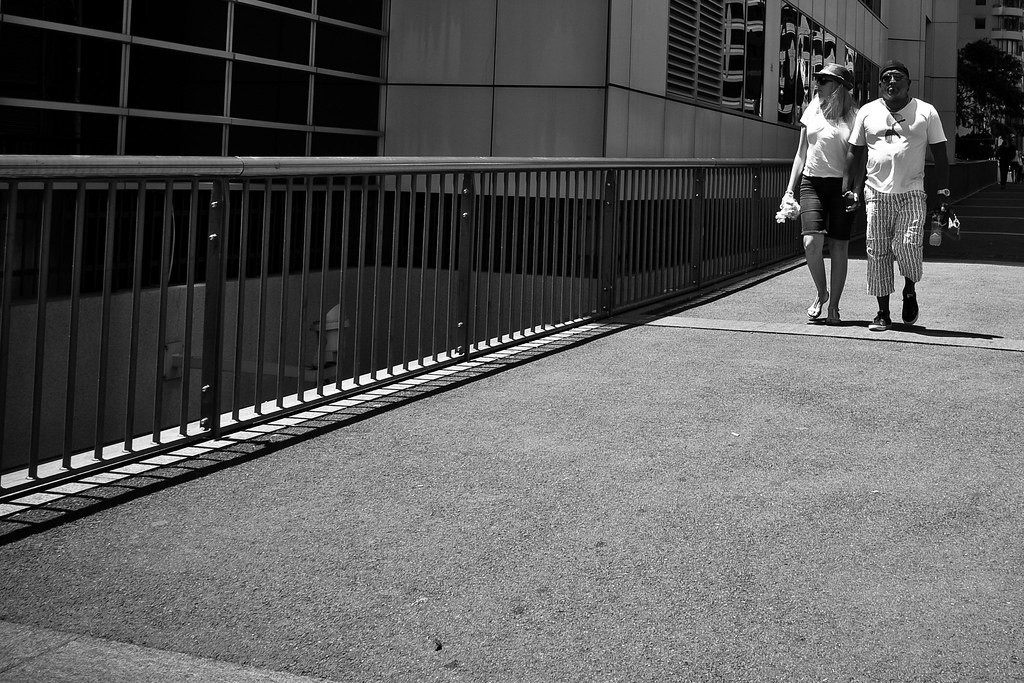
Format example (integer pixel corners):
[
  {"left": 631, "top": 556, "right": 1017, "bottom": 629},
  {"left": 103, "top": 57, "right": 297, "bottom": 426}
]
[
  {"left": 816, "top": 77, "right": 834, "bottom": 86},
  {"left": 881, "top": 76, "right": 907, "bottom": 81}
]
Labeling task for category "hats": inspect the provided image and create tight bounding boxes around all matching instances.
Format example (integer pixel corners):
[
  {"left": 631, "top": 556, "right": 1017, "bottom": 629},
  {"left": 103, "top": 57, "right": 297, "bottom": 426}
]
[
  {"left": 813, "top": 63, "right": 854, "bottom": 90},
  {"left": 880, "top": 59, "right": 909, "bottom": 80}
]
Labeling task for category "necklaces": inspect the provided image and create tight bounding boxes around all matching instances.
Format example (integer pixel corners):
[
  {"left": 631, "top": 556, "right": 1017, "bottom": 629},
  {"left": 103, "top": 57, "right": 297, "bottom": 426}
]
[{"left": 884, "top": 100, "right": 907, "bottom": 111}]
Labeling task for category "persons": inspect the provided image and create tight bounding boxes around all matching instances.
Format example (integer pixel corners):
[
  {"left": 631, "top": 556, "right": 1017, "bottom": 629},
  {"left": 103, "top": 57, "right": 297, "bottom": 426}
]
[
  {"left": 996, "top": 140, "right": 1023, "bottom": 188},
  {"left": 781, "top": 63, "right": 868, "bottom": 325},
  {"left": 841, "top": 60, "right": 952, "bottom": 329}
]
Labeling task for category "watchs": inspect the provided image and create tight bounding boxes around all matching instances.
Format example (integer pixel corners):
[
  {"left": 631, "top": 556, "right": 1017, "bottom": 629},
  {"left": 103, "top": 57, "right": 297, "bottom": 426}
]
[{"left": 938, "top": 189, "right": 952, "bottom": 196}]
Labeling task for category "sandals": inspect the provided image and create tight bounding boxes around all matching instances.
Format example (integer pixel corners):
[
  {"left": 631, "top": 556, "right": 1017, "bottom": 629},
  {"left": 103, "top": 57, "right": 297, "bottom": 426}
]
[
  {"left": 826, "top": 305, "right": 842, "bottom": 324},
  {"left": 807, "top": 292, "right": 829, "bottom": 319}
]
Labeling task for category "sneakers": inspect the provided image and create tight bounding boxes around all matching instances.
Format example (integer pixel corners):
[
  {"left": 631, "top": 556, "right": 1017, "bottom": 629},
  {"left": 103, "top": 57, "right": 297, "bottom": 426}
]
[
  {"left": 902, "top": 287, "right": 918, "bottom": 325},
  {"left": 868, "top": 310, "right": 892, "bottom": 330}
]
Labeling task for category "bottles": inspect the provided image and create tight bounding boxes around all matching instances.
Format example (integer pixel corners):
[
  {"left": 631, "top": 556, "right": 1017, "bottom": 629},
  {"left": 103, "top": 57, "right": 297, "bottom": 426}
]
[{"left": 929, "top": 211, "right": 942, "bottom": 246}]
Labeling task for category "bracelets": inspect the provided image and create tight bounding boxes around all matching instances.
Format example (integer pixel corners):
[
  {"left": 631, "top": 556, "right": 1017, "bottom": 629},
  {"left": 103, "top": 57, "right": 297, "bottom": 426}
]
[
  {"left": 853, "top": 186, "right": 861, "bottom": 190},
  {"left": 784, "top": 190, "right": 795, "bottom": 194}
]
